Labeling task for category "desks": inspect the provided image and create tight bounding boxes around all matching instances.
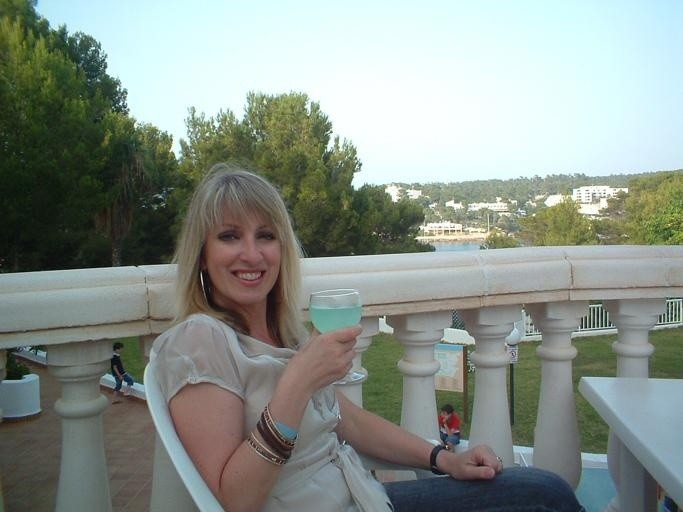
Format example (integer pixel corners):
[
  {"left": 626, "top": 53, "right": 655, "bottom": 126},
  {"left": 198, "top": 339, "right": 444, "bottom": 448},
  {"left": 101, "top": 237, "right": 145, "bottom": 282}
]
[{"left": 577, "top": 377, "right": 682, "bottom": 512}]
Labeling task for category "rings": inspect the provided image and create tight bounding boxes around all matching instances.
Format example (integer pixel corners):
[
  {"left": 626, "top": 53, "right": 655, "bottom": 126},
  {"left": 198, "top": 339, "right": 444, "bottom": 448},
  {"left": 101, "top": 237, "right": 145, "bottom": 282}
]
[{"left": 494, "top": 456, "right": 504, "bottom": 463}]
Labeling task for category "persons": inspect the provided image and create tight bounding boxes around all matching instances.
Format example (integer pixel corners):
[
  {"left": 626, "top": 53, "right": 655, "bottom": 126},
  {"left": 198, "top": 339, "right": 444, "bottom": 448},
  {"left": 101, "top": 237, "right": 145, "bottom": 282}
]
[
  {"left": 153, "top": 163, "right": 586, "bottom": 512},
  {"left": 110, "top": 343, "right": 135, "bottom": 405},
  {"left": 437, "top": 404, "right": 462, "bottom": 447}
]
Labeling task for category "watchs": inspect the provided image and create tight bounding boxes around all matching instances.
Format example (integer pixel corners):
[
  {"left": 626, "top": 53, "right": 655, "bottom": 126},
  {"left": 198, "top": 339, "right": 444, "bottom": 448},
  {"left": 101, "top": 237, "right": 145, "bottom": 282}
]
[{"left": 430, "top": 443, "right": 449, "bottom": 478}]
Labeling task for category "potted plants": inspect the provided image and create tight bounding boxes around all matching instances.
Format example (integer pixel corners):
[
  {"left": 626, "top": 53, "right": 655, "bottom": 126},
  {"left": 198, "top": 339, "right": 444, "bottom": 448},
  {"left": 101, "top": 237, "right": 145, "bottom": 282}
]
[{"left": 0, "top": 347, "right": 43, "bottom": 419}]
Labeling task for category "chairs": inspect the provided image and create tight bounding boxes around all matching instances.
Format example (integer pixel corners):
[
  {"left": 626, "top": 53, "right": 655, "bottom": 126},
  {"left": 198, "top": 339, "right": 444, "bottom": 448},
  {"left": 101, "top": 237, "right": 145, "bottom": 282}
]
[{"left": 141, "top": 359, "right": 227, "bottom": 510}]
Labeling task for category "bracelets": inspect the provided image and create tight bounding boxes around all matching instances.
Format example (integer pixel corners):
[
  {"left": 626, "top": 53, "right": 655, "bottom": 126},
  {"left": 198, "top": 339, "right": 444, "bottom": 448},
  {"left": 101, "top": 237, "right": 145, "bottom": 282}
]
[{"left": 245, "top": 401, "right": 300, "bottom": 467}]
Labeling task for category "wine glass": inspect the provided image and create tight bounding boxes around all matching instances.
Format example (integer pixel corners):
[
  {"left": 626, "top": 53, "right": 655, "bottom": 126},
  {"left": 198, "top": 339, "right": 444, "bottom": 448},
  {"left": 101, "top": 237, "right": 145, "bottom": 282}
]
[{"left": 307, "top": 288, "right": 369, "bottom": 388}]
